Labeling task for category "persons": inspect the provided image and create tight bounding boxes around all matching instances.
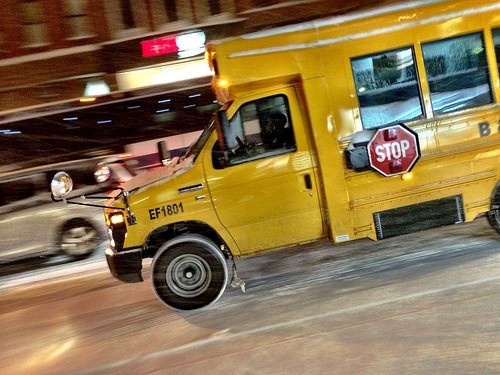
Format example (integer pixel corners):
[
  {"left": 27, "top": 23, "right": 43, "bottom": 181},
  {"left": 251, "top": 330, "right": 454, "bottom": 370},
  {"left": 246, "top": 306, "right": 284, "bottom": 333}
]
[{"left": 256, "top": 108, "right": 291, "bottom": 152}]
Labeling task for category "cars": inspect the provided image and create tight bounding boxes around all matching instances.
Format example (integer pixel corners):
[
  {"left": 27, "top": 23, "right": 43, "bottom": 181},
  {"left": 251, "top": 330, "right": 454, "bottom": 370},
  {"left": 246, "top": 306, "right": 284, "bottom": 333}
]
[{"left": 0, "top": 176, "right": 120, "bottom": 265}]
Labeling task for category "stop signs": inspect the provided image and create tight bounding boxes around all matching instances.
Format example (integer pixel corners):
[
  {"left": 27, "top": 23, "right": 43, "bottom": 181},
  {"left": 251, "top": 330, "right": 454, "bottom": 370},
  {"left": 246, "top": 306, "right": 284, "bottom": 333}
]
[{"left": 368, "top": 125, "right": 421, "bottom": 178}]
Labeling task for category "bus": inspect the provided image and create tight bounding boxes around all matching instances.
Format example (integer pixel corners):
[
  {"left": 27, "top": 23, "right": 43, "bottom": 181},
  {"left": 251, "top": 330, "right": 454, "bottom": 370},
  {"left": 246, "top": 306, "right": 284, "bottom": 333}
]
[
  {"left": 0, "top": 76, "right": 220, "bottom": 176},
  {"left": 47, "top": 0, "right": 499, "bottom": 312}
]
